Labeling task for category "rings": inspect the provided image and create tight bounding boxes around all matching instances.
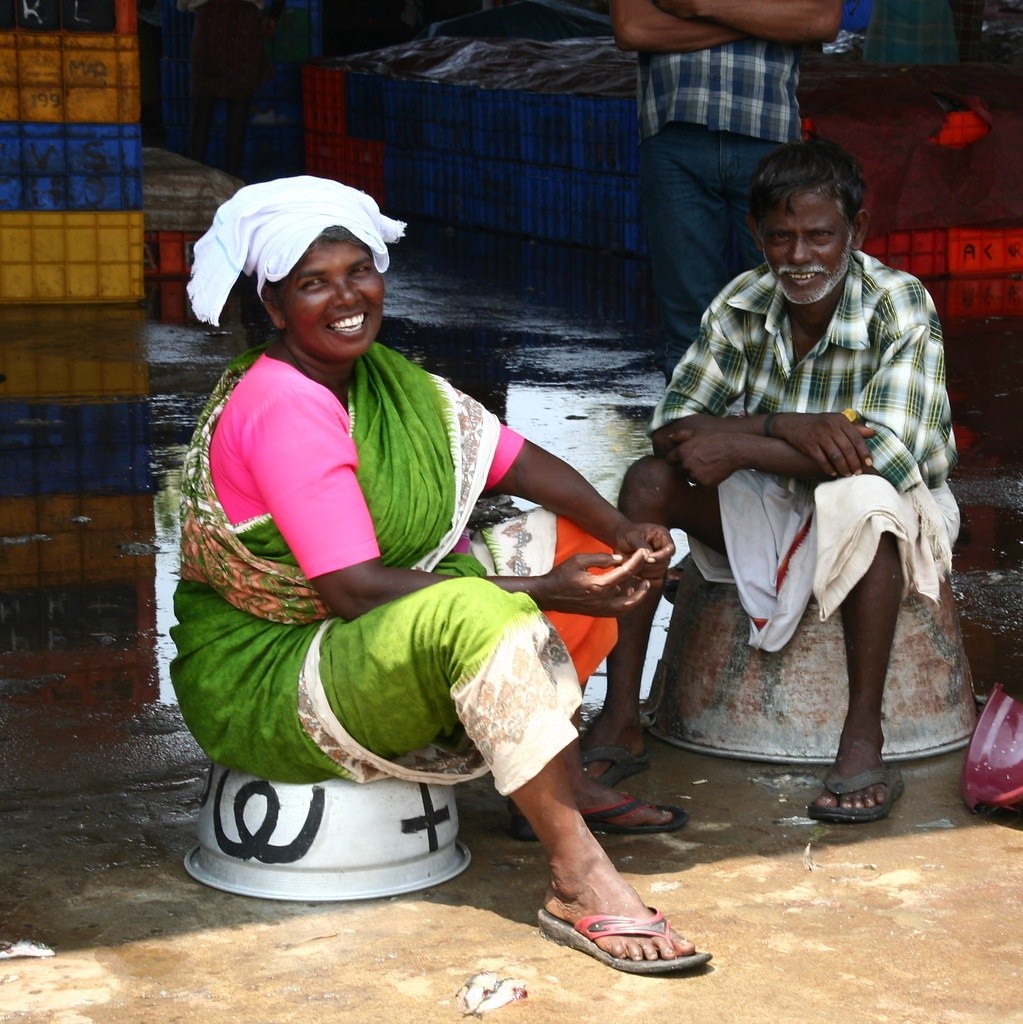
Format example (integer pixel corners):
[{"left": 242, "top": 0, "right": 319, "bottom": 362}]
[{"left": 615, "top": 585, "right": 621, "bottom": 592}]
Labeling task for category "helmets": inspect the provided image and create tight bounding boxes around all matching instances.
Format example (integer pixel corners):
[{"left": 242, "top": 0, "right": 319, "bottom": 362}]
[{"left": 962, "top": 683, "right": 1022, "bottom": 814}]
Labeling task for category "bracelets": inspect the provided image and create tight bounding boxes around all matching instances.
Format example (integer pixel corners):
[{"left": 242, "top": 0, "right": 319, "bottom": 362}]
[{"left": 764, "top": 412, "right": 784, "bottom": 437}]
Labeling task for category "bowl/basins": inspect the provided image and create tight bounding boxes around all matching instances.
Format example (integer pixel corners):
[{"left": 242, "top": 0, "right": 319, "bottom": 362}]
[{"left": 182, "top": 755, "right": 472, "bottom": 902}]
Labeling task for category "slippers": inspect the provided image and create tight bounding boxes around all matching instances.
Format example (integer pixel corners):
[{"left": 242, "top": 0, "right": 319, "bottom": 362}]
[
  {"left": 580, "top": 746, "right": 651, "bottom": 786},
  {"left": 538, "top": 906, "right": 713, "bottom": 972},
  {"left": 808, "top": 765, "right": 903, "bottom": 821},
  {"left": 507, "top": 791, "right": 690, "bottom": 841}
]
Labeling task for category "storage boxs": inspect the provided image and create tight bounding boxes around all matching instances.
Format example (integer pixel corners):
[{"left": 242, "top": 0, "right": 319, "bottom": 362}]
[{"left": 0, "top": 0, "right": 1023, "bottom": 590}]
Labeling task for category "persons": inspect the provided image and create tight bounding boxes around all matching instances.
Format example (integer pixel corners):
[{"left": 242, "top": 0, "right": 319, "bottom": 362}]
[
  {"left": 611, "top": 0, "right": 841, "bottom": 378},
  {"left": 169, "top": 174, "right": 714, "bottom": 973},
  {"left": 578, "top": 142, "right": 960, "bottom": 824}
]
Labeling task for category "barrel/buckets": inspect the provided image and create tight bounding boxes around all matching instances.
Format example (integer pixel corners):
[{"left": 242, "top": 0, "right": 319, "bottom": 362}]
[{"left": 646, "top": 551, "right": 977, "bottom": 764}]
